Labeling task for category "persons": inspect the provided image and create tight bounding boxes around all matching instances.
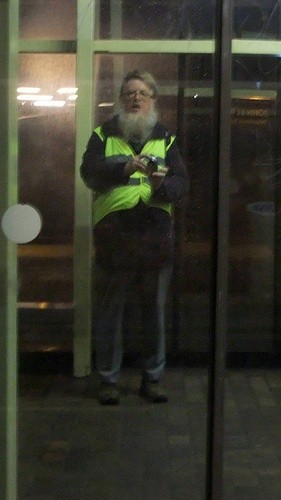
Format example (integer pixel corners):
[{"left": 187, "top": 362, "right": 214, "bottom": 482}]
[{"left": 78, "top": 70, "right": 189, "bottom": 403}]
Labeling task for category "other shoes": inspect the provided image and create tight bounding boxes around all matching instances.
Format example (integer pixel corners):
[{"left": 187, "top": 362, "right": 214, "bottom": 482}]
[
  {"left": 138, "top": 382, "right": 168, "bottom": 403},
  {"left": 97, "top": 385, "right": 121, "bottom": 406}
]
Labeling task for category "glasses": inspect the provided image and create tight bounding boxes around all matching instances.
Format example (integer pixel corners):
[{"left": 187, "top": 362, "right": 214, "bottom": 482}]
[{"left": 120, "top": 89, "right": 153, "bottom": 99}]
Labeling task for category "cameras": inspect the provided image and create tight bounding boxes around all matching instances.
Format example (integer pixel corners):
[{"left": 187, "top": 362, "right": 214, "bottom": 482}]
[{"left": 147, "top": 158, "right": 157, "bottom": 176}]
